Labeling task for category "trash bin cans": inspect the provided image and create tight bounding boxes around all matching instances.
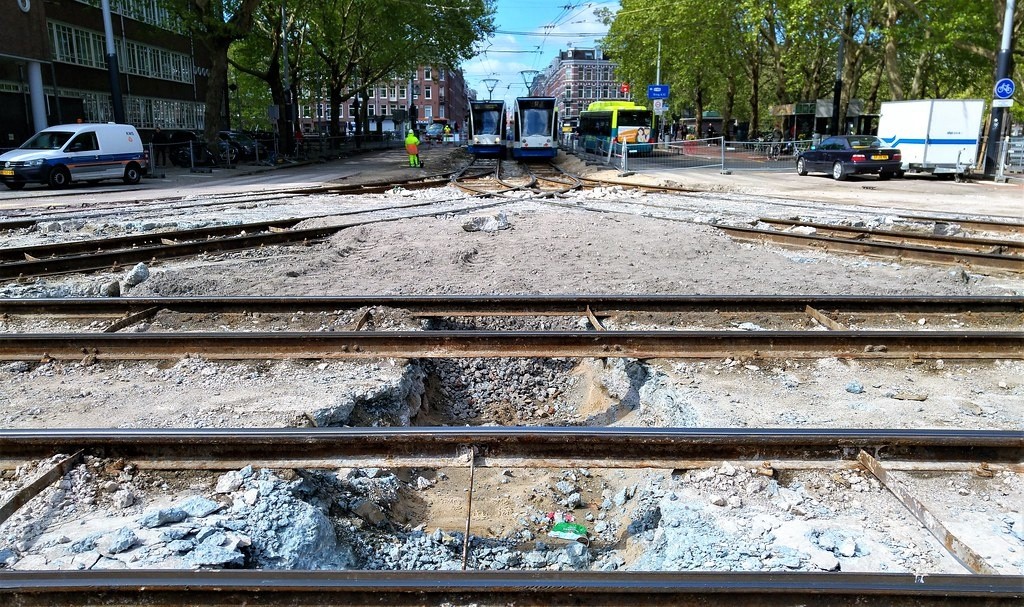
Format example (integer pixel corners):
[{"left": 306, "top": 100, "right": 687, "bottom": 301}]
[
  {"left": 665, "top": 134, "right": 671, "bottom": 148},
  {"left": 812, "top": 133, "right": 820, "bottom": 145}
]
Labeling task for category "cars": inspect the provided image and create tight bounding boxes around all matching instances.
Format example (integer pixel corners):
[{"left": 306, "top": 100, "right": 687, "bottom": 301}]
[
  {"left": 252, "top": 130, "right": 282, "bottom": 153},
  {"left": 796, "top": 134, "right": 902, "bottom": 181},
  {"left": 217, "top": 131, "right": 267, "bottom": 162}
]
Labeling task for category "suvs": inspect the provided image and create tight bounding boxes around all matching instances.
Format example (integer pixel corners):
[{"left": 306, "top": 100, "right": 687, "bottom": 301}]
[{"left": 168, "top": 130, "right": 239, "bottom": 168}]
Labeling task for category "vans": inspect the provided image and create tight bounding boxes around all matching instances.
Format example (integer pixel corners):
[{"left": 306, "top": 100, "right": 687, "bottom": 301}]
[{"left": 0, "top": 119, "right": 147, "bottom": 191}]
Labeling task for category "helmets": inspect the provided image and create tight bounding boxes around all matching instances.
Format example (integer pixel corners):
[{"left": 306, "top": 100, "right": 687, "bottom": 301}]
[
  {"left": 446, "top": 125, "right": 448, "bottom": 127},
  {"left": 409, "top": 129, "right": 413, "bottom": 134}
]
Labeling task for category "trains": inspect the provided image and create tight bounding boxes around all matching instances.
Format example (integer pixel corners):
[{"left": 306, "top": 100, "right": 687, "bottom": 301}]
[
  {"left": 464, "top": 99, "right": 506, "bottom": 156},
  {"left": 513, "top": 96, "right": 559, "bottom": 160}
]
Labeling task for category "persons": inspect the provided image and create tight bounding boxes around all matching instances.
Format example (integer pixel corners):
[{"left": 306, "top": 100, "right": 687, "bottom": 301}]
[
  {"left": 444, "top": 125, "right": 451, "bottom": 134},
  {"left": 454, "top": 122, "right": 459, "bottom": 132},
  {"left": 705, "top": 123, "right": 716, "bottom": 146},
  {"left": 746, "top": 125, "right": 792, "bottom": 159},
  {"left": 637, "top": 128, "right": 649, "bottom": 142},
  {"left": 405, "top": 129, "right": 420, "bottom": 167},
  {"left": 152, "top": 127, "right": 169, "bottom": 166},
  {"left": 674, "top": 123, "right": 688, "bottom": 142}
]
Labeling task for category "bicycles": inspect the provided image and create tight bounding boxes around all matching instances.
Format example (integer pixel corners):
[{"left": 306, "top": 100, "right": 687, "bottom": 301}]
[{"left": 766, "top": 137, "right": 781, "bottom": 161}]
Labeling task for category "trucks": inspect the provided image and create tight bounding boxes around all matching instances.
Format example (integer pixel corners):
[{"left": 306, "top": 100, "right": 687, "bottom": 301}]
[{"left": 425, "top": 118, "right": 451, "bottom": 141}]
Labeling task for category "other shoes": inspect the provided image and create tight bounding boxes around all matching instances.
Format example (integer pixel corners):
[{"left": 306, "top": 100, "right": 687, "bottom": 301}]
[
  {"left": 708, "top": 144, "right": 711, "bottom": 147},
  {"left": 416, "top": 164, "right": 420, "bottom": 167},
  {"left": 411, "top": 164, "right": 415, "bottom": 167}
]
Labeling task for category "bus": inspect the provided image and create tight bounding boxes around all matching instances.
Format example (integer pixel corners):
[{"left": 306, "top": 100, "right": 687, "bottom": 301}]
[{"left": 577, "top": 100, "right": 656, "bottom": 157}]
